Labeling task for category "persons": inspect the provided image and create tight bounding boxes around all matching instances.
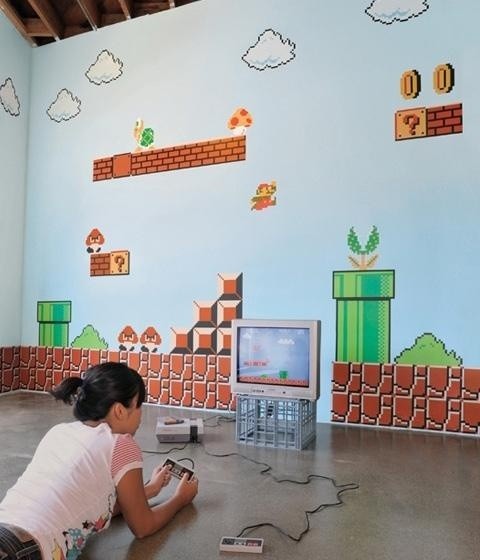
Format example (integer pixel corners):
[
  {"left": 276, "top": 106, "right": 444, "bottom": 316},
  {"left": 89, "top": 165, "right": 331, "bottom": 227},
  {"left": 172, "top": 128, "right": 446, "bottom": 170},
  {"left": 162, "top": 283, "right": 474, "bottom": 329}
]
[{"left": 1, "top": 362, "right": 199, "bottom": 560}]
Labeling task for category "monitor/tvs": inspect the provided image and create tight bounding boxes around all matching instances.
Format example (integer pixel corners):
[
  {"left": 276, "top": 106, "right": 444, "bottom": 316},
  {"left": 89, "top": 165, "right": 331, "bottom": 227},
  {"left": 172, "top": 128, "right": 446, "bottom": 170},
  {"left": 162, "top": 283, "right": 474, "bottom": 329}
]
[{"left": 231, "top": 319, "right": 321, "bottom": 400}]
